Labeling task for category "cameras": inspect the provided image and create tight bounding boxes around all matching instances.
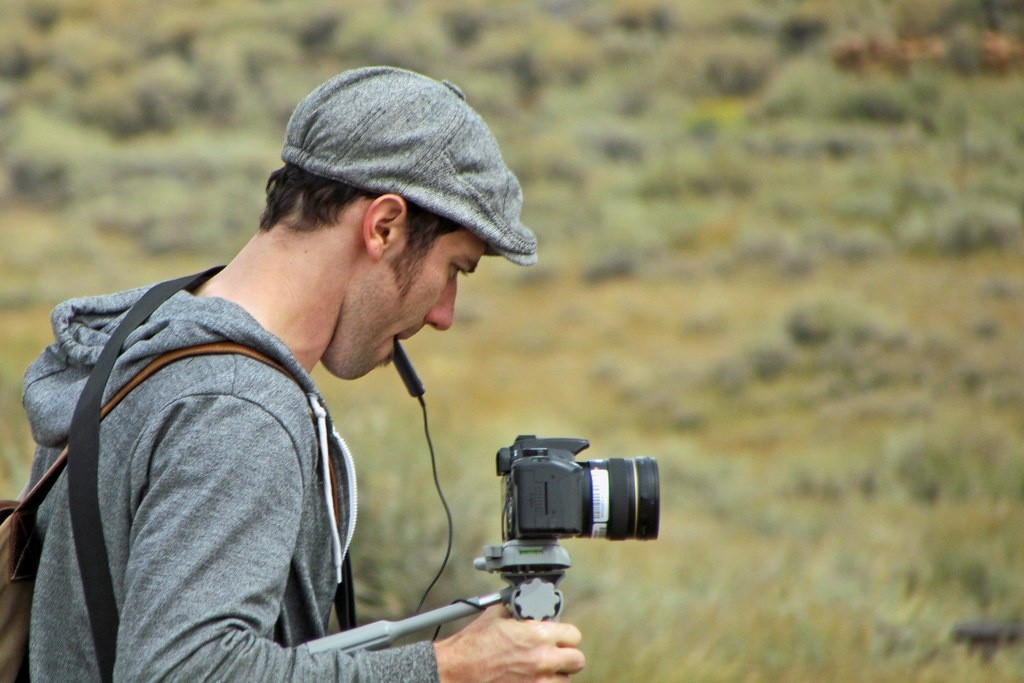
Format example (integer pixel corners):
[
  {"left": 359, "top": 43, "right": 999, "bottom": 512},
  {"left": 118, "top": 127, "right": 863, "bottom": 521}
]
[{"left": 496, "top": 434, "right": 661, "bottom": 541}]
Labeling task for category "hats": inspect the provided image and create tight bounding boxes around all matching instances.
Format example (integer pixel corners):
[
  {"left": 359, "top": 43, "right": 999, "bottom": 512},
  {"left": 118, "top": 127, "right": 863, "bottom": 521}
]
[{"left": 281, "top": 65, "right": 538, "bottom": 266}]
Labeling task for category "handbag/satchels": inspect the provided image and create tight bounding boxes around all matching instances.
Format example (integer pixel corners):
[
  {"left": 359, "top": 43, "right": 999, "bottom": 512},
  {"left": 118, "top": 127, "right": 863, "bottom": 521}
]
[{"left": 0, "top": 499, "right": 40, "bottom": 682}]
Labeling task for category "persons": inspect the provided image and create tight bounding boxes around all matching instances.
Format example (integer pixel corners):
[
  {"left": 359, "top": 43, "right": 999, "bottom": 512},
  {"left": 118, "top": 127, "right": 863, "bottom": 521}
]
[{"left": 17, "top": 67, "right": 585, "bottom": 683}]
